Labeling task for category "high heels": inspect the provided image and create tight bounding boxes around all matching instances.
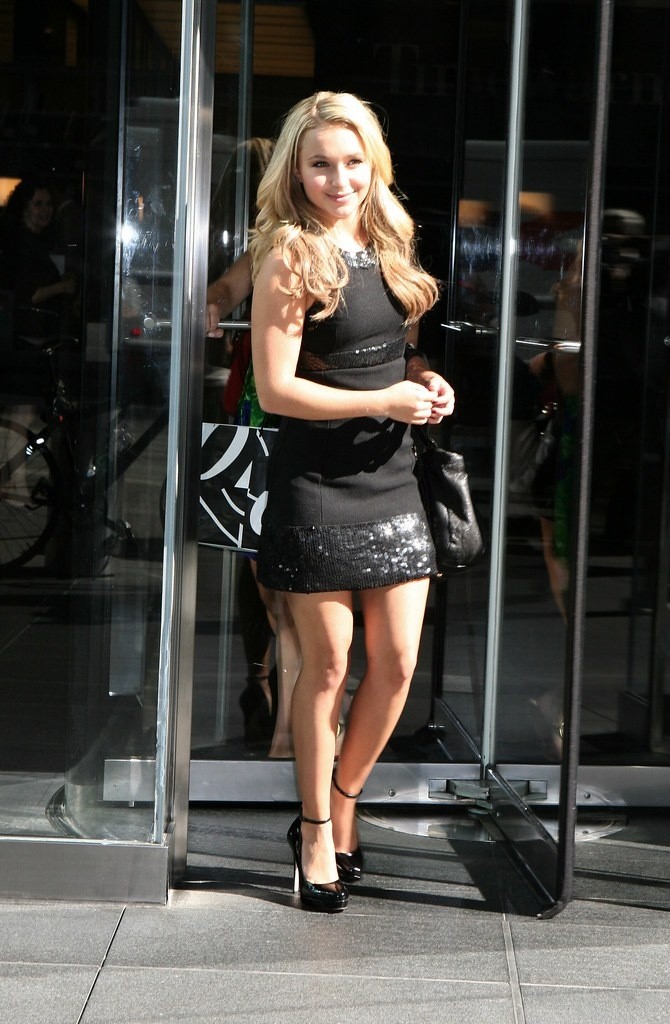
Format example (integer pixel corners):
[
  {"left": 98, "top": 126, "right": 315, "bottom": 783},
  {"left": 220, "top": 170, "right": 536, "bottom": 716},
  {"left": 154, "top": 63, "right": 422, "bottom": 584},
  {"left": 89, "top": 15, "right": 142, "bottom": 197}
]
[
  {"left": 286, "top": 808, "right": 348, "bottom": 914},
  {"left": 331, "top": 769, "right": 365, "bottom": 882}
]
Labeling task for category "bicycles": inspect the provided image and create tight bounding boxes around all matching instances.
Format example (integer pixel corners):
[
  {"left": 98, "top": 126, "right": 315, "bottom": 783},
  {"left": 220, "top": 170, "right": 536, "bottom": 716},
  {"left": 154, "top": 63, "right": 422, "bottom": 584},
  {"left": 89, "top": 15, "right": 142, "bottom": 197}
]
[{"left": 0, "top": 381, "right": 167, "bottom": 579}]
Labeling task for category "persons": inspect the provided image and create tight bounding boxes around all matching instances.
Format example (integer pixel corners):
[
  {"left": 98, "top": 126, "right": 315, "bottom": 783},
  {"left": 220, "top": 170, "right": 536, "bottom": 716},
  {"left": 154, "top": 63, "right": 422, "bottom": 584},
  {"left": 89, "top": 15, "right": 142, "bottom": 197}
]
[
  {"left": 2, "top": 184, "right": 77, "bottom": 508},
  {"left": 204, "top": 90, "right": 455, "bottom": 914},
  {"left": 529, "top": 239, "right": 581, "bottom": 626}
]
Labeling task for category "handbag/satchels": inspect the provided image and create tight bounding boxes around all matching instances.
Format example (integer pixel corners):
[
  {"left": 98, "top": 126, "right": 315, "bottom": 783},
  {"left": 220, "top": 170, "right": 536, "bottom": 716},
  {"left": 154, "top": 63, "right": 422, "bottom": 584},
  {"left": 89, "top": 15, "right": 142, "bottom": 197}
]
[
  {"left": 504, "top": 403, "right": 560, "bottom": 502},
  {"left": 414, "top": 445, "right": 480, "bottom": 569}
]
